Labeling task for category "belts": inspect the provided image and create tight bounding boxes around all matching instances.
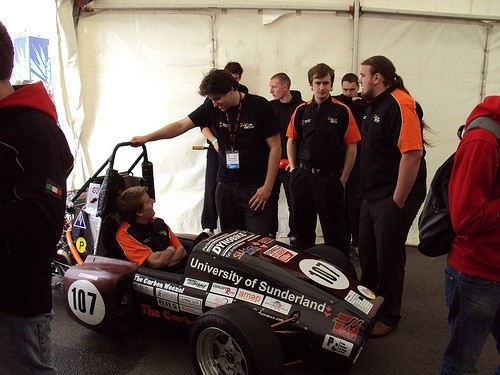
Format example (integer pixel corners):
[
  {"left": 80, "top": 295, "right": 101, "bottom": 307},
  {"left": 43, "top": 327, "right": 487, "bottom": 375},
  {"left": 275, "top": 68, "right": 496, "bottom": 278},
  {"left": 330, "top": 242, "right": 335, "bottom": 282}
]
[{"left": 299, "top": 162, "right": 342, "bottom": 177}]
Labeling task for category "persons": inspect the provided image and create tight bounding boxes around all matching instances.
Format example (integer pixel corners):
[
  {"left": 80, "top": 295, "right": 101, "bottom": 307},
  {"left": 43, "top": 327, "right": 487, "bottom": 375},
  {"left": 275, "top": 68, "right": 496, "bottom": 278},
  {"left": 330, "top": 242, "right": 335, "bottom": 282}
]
[
  {"left": 201, "top": 62, "right": 248, "bottom": 236},
  {"left": 437, "top": 92, "right": 500, "bottom": 375},
  {"left": 0, "top": 20, "right": 75, "bottom": 375},
  {"left": 131, "top": 68, "right": 281, "bottom": 241},
  {"left": 357, "top": 55, "right": 439, "bottom": 337},
  {"left": 114, "top": 184, "right": 187, "bottom": 270},
  {"left": 285, "top": 62, "right": 361, "bottom": 257},
  {"left": 267, "top": 72, "right": 308, "bottom": 246}
]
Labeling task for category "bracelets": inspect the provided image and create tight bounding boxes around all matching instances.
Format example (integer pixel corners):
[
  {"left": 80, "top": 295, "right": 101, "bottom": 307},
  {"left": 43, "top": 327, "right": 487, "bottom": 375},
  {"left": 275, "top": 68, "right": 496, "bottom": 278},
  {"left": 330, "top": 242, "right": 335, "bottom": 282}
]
[
  {"left": 211, "top": 137, "right": 217, "bottom": 146},
  {"left": 167, "top": 246, "right": 175, "bottom": 253}
]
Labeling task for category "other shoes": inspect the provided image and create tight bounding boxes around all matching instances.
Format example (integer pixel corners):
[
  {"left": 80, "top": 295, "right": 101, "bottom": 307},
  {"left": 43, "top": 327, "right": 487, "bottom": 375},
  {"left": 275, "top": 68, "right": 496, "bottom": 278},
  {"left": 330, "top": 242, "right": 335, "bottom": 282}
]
[
  {"left": 348, "top": 244, "right": 359, "bottom": 258},
  {"left": 370, "top": 320, "right": 396, "bottom": 338},
  {"left": 202, "top": 227, "right": 214, "bottom": 237}
]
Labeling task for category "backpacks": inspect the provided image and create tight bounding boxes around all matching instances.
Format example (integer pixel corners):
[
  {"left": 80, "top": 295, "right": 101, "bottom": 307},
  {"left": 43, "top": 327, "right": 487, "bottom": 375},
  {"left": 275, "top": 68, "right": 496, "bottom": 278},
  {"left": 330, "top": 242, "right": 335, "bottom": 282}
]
[{"left": 417, "top": 117, "right": 500, "bottom": 258}]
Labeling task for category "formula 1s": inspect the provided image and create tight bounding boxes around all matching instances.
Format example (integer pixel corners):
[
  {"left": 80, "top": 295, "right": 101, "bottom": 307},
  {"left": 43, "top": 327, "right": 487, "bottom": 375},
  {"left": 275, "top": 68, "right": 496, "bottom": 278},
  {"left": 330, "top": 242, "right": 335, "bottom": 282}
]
[{"left": 52, "top": 141, "right": 389, "bottom": 374}]
